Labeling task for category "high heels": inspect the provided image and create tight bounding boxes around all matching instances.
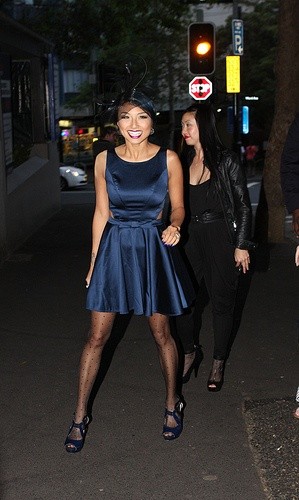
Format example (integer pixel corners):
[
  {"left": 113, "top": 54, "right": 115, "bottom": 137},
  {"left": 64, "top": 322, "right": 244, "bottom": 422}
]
[
  {"left": 207, "top": 359, "right": 225, "bottom": 391},
  {"left": 177, "top": 348, "right": 203, "bottom": 383}
]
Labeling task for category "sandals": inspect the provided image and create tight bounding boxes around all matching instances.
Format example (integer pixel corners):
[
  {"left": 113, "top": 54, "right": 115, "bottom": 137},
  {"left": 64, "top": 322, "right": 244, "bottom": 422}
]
[
  {"left": 64, "top": 412, "right": 91, "bottom": 452},
  {"left": 161, "top": 393, "right": 186, "bottom": 439}
]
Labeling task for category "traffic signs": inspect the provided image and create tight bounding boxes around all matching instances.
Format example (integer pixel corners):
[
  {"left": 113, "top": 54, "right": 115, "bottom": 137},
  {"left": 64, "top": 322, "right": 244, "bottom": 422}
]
[{"left": 231, "top": 20, "right": 244, "bottom": 56}]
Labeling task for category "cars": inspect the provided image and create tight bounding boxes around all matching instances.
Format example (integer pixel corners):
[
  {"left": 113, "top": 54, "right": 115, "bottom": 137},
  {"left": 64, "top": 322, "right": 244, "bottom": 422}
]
[{"left": 59, "top": 165, "right": 90, "bottom": 191}]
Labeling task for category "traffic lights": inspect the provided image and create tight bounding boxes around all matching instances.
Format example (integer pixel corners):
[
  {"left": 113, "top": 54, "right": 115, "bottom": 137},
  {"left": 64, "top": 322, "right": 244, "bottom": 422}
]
[{"left": 186, "top": 22, "right": 217, "bottom": 77}]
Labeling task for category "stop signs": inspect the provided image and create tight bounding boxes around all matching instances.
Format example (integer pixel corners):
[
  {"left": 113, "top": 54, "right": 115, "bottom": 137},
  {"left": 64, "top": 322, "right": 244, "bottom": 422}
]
[{"left": 188, "top": 77, "right": 214, "bottom": 101}]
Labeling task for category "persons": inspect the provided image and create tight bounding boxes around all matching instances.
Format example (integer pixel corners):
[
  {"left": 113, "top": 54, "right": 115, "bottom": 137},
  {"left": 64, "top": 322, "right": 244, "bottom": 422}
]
[
  {"left": 166, "top": 103, "right": 258, "bottom": 392},
  {"left": 93, "top": 126, "right": 117, "bottom": 158},
  {"left": 279, "top": 113, "right": 299, "bottom": 235},
  {"left": 64, "top": 88, "right": 196, "bottom": 453}
]
